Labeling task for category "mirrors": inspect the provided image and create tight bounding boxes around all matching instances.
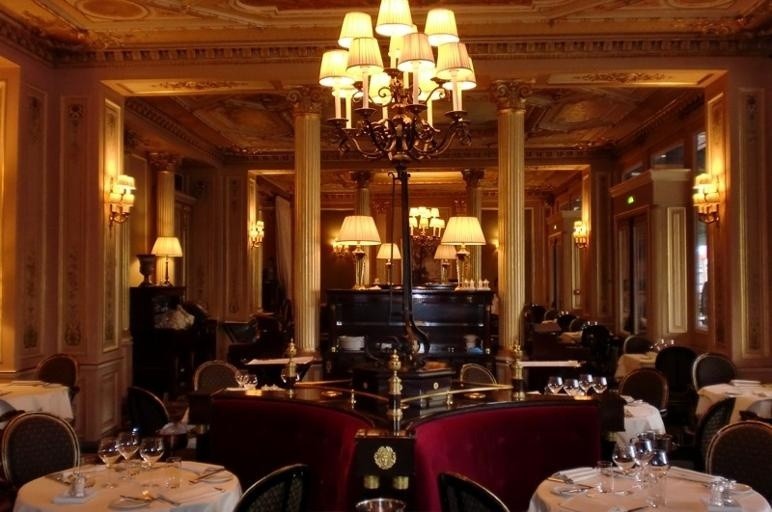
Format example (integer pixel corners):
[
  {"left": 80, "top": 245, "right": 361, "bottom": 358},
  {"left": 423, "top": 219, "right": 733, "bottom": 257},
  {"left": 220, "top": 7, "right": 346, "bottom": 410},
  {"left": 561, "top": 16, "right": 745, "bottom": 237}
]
[{"left": 370, "top": 194, "right": 457, "bottom": 289}]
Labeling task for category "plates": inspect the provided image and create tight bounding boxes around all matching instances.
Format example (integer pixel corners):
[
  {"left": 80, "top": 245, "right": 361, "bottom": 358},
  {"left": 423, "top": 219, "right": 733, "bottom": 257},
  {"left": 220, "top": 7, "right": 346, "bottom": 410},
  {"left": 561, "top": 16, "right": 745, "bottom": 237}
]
[
  {"left": 552, "top": 485, "right": 588, "bottom": 495},
  {"left": 203, "top": 469, "right": 233, "bottom": 482},
  {"left": 340, "top": 335, "right": 365, "bottom": 350},
  {"left": 731, "top": 482, "right": 751, "bottom": 494},
  {"left": 111, "top": 496, "right": 150, "bottom": 508},
  {"left": 425, "top": 282, "right": 457, "bottom": 287}
]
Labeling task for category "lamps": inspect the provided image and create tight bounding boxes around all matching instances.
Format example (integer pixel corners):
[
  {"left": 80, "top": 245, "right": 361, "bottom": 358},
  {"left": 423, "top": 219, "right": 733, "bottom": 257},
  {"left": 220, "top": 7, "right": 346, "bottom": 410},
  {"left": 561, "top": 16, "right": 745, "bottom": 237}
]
[
  {"left": 335, "top": 216, "right": 381, "bottom": 292},
  {"left": 440, "top": 216, "right": 487, "bottom": 292},
  {"left": 376, "top": 243, "right": 402, "bottom": 286},
  {"left": 318, "top": 0, "right": 478, "bottom": 409},
  {"left": 328, "top": 240, "right": 349, "bottom": 258},
  {"left": 692, "top": 173, "right": 720, "bottom": 225},
  {"left": 572, "top": 220, "right": 587, "bottom": 250},
  {"left": 409, "top": 206, "right": 446, "bottom": 284},
  {"left": 434, "top": 244, "right": 457, "bottom": 285},
  {"left": 151, "top": 236, "right": 183, "bottom": 287},
  {"left": 109, "top": 174, "right": 137, "bottom": 227},
  {"left": 251, "top": 221, "right": 264, "bottom": 249}
]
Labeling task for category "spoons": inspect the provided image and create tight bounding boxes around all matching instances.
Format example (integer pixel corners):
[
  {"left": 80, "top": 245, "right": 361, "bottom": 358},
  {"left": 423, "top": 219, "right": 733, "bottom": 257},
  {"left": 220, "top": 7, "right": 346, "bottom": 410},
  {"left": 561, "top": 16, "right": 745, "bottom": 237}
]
[{"left": 143, "top": 490, "right": 181, "bottom": 506}]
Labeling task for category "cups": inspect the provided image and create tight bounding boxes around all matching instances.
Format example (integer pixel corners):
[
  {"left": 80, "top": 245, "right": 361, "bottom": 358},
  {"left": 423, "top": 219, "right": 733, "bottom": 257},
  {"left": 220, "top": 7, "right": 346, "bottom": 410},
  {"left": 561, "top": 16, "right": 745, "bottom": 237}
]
[
  {"left": 596, "top": 460, "right": 613, "bottom": 494},
  {"left": 464, "top": 334, "right": 478, "bottom": 350},
  {"left": 166, "top": 456, "right": 183, "bottom": 488},
  {"left": 236, "top": 369, "right": 249, "bottom": 389},
  {"left": 80, "top": 456, "right": 97, "bottom": 487},
  {"left": 608, "top": 384, "right": 620, "bottom": 394},
  {"left": 646, "top": 338, "right": 675, "bottom": 358},
  {"left": 245, "top": 374, "right": 257, "bottom": 389}
]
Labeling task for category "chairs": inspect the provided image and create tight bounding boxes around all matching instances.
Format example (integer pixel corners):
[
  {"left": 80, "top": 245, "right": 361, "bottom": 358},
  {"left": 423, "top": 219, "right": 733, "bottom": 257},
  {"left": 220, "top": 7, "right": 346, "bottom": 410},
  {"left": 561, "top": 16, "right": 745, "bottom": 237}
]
[
  {"left": 437, "top": 472, "right": 511, "bottom": 512},
  {"left": 1, "top": 412, "right": 80, "bottom": 492},
  {"left": 192, "top": 360, "right": 243, "bottom": 393},
  {"left": 223, "top": 297, "right": 294, "bottom": 389},
  {"left": 231, "top": 463, "right": 309, "bottom": 512},
  {"left": 33, "top": 353, "right": 80, "bottom": 401},
  {"left": 527, "top": 304, "right": 771, "bottom": 508},
  {"left": 127, "top": 386, "right": 170, "bottom": 436}
]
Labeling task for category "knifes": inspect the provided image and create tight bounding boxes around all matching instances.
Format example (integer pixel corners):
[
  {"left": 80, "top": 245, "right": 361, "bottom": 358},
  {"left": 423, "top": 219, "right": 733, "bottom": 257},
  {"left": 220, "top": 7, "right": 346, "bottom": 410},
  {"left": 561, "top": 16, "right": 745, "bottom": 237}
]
[
  {"left": 120, "top": 494, "right": 148, "bottom": 503},
  {"left": 560, "top": 487, "right": 594, "bottom": 493}
]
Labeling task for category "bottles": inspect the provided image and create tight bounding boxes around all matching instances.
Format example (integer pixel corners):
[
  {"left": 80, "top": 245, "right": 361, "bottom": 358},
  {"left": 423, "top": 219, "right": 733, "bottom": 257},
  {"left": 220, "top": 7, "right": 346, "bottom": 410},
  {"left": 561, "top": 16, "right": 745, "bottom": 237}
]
[
  {"left": 707, "top": 477, "right": 736, "bottom": 504},
  {"left": 73, "top": 475, "right": 87, "bottom": 497}
]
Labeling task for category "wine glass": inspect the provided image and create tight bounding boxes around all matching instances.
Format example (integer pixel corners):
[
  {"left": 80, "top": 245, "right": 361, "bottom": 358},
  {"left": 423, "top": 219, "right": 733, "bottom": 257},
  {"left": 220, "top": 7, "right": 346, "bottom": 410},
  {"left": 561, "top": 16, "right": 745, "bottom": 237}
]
[
  {"left": 118, "top": 431, "right": 138, "bottom": 480},
  {"left": 100, "top": 437, "right": 119, "bottom": 491},
  {"left": 634, "top": 433, "right": 670, "bottom": 509},
  {"left": 548, "top": 374, "right": 608, "bottom": 400},
  {"left": 141, "top": 437, "right": 163, "bottom": 488},
  {"left": 613, "top": 442, "right": 636, "bottom": 495}
]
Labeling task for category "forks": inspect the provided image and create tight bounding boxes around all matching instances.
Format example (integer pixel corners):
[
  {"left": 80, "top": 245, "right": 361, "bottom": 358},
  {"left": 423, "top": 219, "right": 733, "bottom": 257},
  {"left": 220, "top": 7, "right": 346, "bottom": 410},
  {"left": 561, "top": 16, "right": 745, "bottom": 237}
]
[{"left": 193, "top": 467, "right": 225, "bottom": 483}]
